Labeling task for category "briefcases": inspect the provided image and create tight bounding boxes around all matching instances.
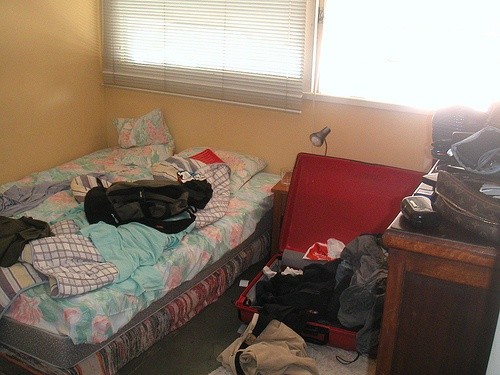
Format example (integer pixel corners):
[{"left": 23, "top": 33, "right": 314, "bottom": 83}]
[{"left": 235, "top": 150, "right": 427, "bottom": 351}]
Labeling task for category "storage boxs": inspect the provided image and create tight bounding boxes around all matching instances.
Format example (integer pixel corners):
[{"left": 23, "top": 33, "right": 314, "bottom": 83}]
[{"left": 234, "top": 152, "right": 427, "bottom": 353}]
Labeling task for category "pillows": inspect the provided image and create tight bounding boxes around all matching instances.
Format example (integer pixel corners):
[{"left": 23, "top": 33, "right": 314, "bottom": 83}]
[
  {"left": 112, "top": 107, "right": 174, "bottom": 167},
  {"left": 179, "top": 148, "right": 268, "bottom": 192}
]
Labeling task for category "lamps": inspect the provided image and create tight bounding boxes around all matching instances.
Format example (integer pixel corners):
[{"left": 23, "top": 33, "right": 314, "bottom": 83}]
[{"left": 310, "top": 127, "right": 331, "bottom": 156}]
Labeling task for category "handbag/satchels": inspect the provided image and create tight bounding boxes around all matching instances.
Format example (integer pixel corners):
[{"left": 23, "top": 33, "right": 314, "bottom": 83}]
[{"left": 433, "top": 170, "right": 500, "bottom": 242}]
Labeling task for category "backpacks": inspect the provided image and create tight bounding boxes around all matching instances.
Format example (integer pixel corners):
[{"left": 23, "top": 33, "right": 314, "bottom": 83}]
[{"left": 105, "top": 179, "right": 198, "bottom": 234}]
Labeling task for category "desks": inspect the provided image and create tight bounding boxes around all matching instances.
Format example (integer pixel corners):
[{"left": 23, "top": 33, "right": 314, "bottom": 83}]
[{"left": 374, "top": 160, "right": 500, "bottom": 375}]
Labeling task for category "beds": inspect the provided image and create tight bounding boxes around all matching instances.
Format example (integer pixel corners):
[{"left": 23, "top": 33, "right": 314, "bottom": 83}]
[{"left": 0, "top": 147, "right": 280, "bottom": 375}]
